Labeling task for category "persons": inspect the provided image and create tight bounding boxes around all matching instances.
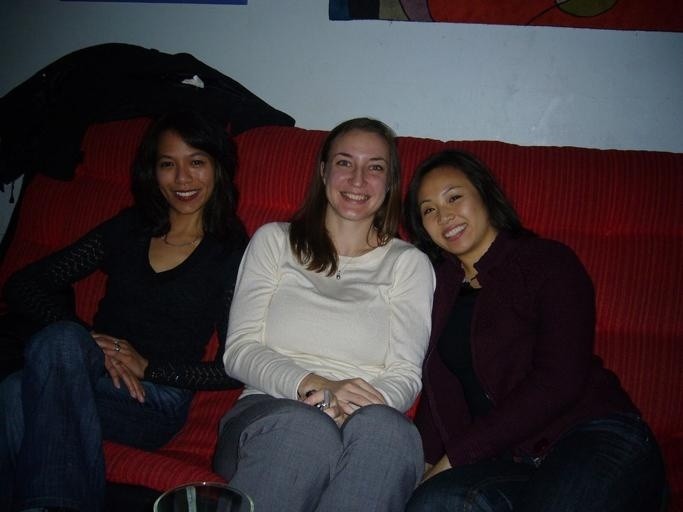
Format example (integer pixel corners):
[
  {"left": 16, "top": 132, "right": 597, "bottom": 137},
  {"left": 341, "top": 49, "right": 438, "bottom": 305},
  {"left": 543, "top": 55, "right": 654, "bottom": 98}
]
[
  {"left": 211, "top": 113, "right": 438, "bottom": 512},
  {"left": 0, "top": 99, "right": 251, "bottom": 512},
  {"left": 401, "top": 143, "right": 671, "bottom": 511}
]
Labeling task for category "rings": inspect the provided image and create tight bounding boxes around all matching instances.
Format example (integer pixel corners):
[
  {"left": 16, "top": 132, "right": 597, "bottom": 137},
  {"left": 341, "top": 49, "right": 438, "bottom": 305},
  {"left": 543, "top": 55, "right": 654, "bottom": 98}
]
[
  {"left": 114, "top": 343, "right": 120, "bottom": 351},
  {"left": 112, "top": 336, "right": 119, "bottom": 345}
]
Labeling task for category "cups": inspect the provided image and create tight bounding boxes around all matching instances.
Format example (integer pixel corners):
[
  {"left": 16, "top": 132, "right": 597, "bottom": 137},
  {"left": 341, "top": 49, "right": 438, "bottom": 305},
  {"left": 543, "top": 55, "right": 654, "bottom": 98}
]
[{"left": 154, "top": 483, "right": 254, "bottom": 512}]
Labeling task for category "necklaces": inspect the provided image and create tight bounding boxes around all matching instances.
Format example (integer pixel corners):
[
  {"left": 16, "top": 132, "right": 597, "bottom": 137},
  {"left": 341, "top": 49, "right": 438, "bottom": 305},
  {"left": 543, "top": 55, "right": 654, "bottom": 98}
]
[
  {"left": 461, "top": 265, "right": 478, "bottom": 288},
  {"left": 162, "top": 228, "right": 201, "bottom": 250},
  {"left": 329, "top": 240, "right": 364, "bottom": 281}
]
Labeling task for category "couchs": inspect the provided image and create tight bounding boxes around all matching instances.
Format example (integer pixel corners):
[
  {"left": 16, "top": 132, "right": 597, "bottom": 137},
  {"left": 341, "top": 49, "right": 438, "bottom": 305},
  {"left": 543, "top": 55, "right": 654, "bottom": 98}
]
[{"left": 1, "top": 117, "right": 683, "bottom": 511}]
